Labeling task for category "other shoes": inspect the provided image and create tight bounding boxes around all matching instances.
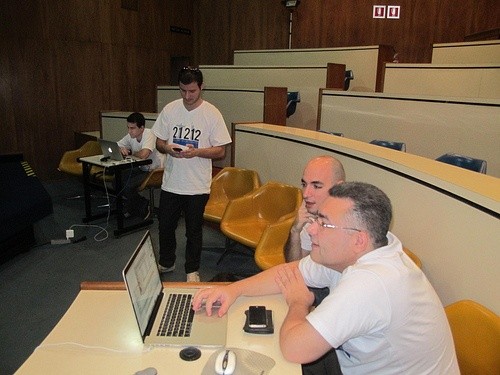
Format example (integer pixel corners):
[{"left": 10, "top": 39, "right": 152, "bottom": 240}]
[
  {"left": 157, "top": 262, "right": 176, "bottom": 273},
  {"left": 186, "top": 271, "right": 201, "bottom": 282}
]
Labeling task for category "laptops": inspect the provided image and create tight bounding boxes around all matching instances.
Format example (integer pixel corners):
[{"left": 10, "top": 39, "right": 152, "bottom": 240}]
[
  {"left": 122, "top": 230, "right": 228, "bottom": 347},
  {"left": 99, "top": 140, "right": 135, "bottom": 160}
]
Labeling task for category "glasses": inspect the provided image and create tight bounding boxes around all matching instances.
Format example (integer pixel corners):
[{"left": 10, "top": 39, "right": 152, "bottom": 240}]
[
  {"left": 180, "top": 65, "right": 200, "bottom": 73},
  {"left": 311, "top": 214, "right": 362, "bottom": 233}
]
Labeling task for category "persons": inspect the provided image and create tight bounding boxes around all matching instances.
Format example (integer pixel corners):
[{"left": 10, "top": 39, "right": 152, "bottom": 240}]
[
  {"left": 192, "top": 182, "right": 460, "bottom": 375},
  {"left": 284, "top": 155, "right": 346, "bottom": 305},
  {"left": 150, "top": 67, "right": 232, "bottom": 282},
  {"left": 112, "top": 113, "right": 161, "bottom": 221}
]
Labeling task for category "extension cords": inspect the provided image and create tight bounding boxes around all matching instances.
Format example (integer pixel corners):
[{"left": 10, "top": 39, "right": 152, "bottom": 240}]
[{"left": 51, "top": 238, "right": 70, "bottom": 244}]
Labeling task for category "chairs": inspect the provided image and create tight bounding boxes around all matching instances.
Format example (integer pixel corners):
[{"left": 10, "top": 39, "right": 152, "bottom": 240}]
[
  {"left": 435, "top": 152, "right": 486, "bottom": 175},
  {"left": 369, "top": 140, "right": 407, "bottom": 153},
  {"left": 344, "top": 70, "right": 354, "bottom": 91},
  {"left": 285, "top": 91, "right": 301, "bottom": 119},
  {"left": 58, "top": 140, "right": 165, "bottom": 219},
  {"left": 200, "top": 167, "right": 500, "bottom": 375}
]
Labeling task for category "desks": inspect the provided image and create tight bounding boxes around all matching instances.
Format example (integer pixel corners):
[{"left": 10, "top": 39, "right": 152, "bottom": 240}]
[
  {"left": 98, "top": 41, "right": 500, "bottom": 319},
  {"left": 13, "top": 281, "right": 303, "bottom": 375},
  {"left": 77, "top": 153, "right": 153, "bottom": 237}
]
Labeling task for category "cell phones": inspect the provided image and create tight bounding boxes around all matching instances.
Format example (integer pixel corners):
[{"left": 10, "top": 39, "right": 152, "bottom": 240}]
[
  {"left": 215, "top": 350, "right": 236, "bottom": 375},
  {"left": 173, "top": 148, "right": 182, "bottom": 152},
  {"left": 248, "top": 306, "right": 267, "bottom": 328}
]
